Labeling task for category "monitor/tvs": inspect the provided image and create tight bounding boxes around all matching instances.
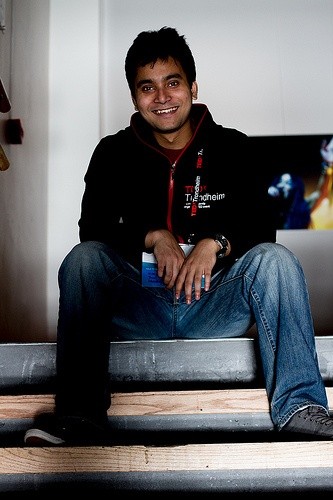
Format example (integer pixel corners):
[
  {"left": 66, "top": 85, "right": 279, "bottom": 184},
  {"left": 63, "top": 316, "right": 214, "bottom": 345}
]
[{"left": 248, "top": 135, "right": 333, "bottom": 230}]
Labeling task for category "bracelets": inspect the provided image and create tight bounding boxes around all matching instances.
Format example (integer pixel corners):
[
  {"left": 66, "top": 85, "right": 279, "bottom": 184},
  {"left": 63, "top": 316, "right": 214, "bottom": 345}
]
[{"left": 209, "top": 234, "right": 228, "bottom": 258}]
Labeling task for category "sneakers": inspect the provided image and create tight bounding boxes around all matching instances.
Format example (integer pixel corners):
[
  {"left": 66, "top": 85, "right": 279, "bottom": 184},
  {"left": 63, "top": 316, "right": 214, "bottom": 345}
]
[
  {"left": 274, "top": 406, "right": 333, "bottom": 441},
  {"left": 24, "top": 407, "right": 112, "bottom": 447}
]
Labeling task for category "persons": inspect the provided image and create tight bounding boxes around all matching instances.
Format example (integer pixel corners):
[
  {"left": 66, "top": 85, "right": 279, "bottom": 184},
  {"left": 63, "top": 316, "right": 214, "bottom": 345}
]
[{"left": 24, "top": 26, "right": 333, "bottom": 447}]
